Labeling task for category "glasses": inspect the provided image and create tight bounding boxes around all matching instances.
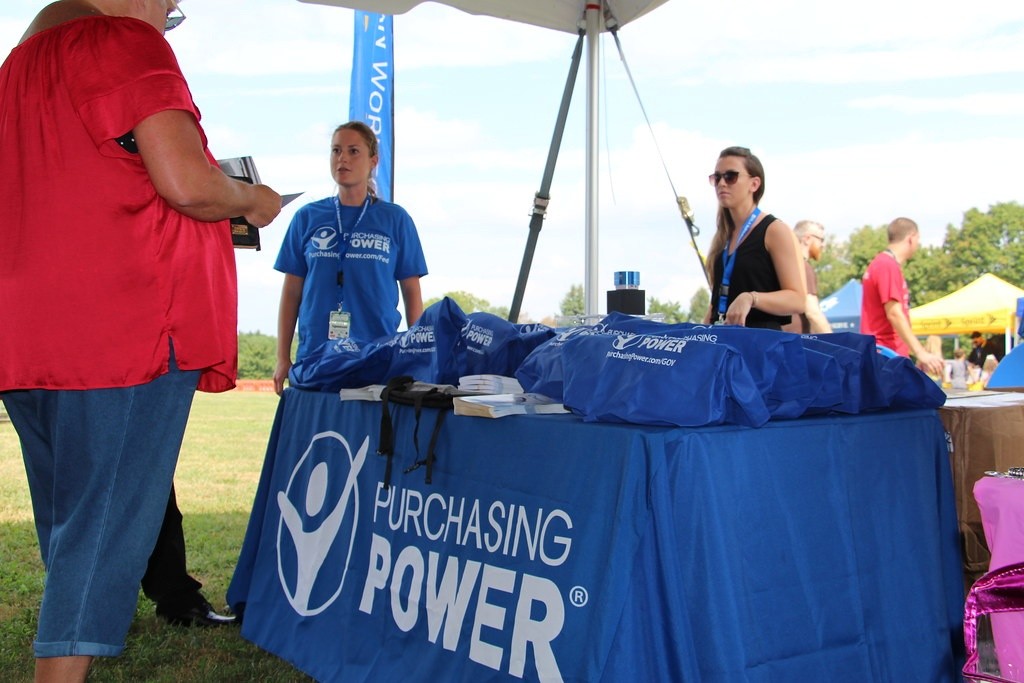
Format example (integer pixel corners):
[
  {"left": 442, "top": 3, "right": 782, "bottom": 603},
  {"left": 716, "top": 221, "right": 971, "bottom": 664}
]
[
  {"left": 164, "top": 0, "right": 186, "bottom": 31},
  {"left": 708, "top": 171, "right": 752, "bottom": 187},
  {"left": 810, "top": 235, "right": 824, "bottom": 243}
]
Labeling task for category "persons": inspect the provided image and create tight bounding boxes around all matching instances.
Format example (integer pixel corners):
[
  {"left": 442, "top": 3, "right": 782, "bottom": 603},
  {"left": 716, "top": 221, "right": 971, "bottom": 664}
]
[
  {"left": 794, "top": 220, "right": 833, "bottom": 333},
  {"left": 273, "top": 121, "right": 429, "bottom": 397},
  {"left": 704, "top": 146, "right": 806, "bottom": 331},
  {"left": 141, "top": 481, "right": 239, "bottom": 627},
  {"left": 861, "top": 217, "right": 945, "bottom": 375},
  {"left": 0, "top": 1, "right": 282, "bottom": 683},
  {"left": 926, "top": 331, "right": 1003, "bottom": 389}
]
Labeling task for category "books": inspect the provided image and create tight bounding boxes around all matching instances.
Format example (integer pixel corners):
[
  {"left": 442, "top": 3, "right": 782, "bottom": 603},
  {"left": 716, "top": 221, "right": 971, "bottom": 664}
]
[
  {"left": 340, "top": 384, "right": 386, "bottom": 400},
  {"left": 452, "top": 374, "right": 571, "bottom": 418}
]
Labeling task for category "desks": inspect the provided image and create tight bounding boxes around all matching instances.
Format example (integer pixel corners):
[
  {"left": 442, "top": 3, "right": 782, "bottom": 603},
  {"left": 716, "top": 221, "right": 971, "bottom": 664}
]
[{"left": 225, "top": 386, "right": 965, "bottom": 683}]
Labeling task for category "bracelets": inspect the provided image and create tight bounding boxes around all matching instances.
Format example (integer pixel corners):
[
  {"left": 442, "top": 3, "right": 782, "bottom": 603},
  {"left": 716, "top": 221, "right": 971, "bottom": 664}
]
[{"left": 749, "top": 292, "right": 757, "bottom": 307}]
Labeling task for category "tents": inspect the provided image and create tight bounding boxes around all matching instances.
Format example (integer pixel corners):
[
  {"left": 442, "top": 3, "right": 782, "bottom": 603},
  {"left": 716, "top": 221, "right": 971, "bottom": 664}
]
[
  {"left": 910, "top": 274, "right": 1024, "bottom": 355},
  {"left": 819, "top": 280, "right": 862, "bottom": 333}
]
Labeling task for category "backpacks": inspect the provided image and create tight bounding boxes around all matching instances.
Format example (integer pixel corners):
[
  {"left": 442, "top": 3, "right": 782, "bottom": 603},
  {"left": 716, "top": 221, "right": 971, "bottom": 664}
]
[{"left": 377, "top": 376, "right": 476, "bottom": 411}]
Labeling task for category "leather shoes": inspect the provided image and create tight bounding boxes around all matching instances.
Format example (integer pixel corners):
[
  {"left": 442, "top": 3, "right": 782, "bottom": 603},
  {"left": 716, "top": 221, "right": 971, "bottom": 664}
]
[{"left": 167, "top": 598, "right": 243, "bottom": 628}]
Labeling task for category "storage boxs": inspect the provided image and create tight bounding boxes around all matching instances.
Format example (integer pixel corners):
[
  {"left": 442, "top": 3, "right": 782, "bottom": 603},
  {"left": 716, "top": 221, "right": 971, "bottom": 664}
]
[{"left": 938, "top": 391, "right": 1024, "bottom": 533}]
